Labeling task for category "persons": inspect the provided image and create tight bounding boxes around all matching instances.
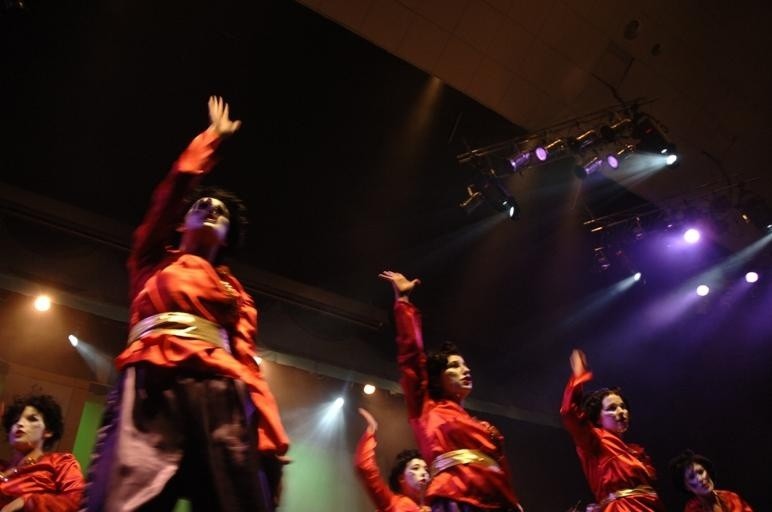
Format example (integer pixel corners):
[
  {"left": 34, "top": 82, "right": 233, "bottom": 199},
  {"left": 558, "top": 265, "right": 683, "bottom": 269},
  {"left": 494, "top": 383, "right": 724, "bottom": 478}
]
[
  {"left": 375, "top": 267, "right": 526, "bottom": 511},
  {"left": 557, "top": 349, "right": 664, "bottom": 511},
  {"left": 1, "top": 392, "right": 86, "bottom": 511},
  {"left": 669, "top": 448, "right": 753, "bottom": 512},
  {"left": 82, "top": 92, "right": 292, "bottom": 511},
  {"left": 352, "top": 404, "right": 437, "bottom": 512}
]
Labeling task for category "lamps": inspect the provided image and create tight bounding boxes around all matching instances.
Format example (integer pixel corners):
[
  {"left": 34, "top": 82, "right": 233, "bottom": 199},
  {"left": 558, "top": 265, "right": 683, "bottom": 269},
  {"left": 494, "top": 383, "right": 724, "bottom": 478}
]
[{"left": 455, "top": 93, "right": 772, "bottom": 295}]
